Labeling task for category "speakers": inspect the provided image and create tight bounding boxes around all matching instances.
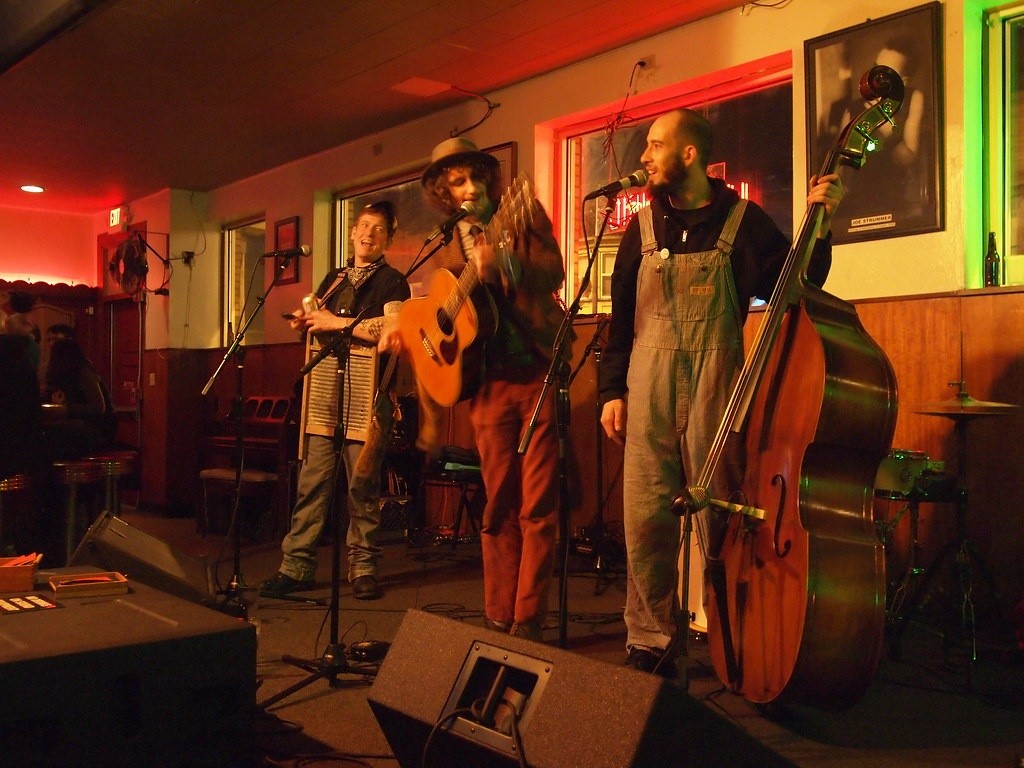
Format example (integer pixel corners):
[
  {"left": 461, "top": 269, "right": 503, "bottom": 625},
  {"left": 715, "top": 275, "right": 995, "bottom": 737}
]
[
  {"left": 64, "top": 509, "right": 221, "bottom": 607},
  {"left": 366, "top": 608, "right": 803, "bottom": 768}
]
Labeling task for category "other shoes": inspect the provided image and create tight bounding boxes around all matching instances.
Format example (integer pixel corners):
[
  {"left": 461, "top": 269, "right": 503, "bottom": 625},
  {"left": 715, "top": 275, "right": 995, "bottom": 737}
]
[
  {"left": 620, "top": 646, "right": 669, "bottom": 675},
  {"left": 487, "top": 618, "right": 542, "bottom": 646}
]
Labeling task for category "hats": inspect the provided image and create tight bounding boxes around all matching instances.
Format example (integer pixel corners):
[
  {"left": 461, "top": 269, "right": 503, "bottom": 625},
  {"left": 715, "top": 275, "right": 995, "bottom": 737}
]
[
  {"left": 420, "top": 138, "right": 502, "bottom": 194},
  {"left": 366, "top": 200, "right": 399, "bottom": 239}
]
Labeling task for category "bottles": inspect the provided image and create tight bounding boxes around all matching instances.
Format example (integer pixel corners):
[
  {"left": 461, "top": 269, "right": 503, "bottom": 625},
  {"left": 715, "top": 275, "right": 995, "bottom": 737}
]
[{"left": 985, "top": 232, "right": 1001, "bottom": 287}]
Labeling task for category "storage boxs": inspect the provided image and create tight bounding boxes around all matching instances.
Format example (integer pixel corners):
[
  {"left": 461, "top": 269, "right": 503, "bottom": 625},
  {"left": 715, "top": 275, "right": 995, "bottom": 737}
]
[
  {"left": 50, "top": 572, "right": 128, "bottom": 598},
  {"left": 0, "top": 553, "right": 43, "bottom": 592}
]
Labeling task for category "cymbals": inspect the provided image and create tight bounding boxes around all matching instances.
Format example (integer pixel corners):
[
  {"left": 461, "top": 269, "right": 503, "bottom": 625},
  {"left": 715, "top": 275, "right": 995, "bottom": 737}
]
[{"left": 904, "top": 395, "right": 1022, "bottom": 420}]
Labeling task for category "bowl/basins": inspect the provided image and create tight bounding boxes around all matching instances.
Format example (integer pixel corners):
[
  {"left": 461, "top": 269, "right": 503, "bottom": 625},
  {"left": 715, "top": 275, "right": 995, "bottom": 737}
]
[{"left": 39, "top": 400, "right": 68, "bottom": 420}]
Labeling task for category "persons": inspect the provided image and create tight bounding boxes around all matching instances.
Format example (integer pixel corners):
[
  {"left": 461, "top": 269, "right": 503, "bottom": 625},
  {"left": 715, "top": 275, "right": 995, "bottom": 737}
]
[
  {"left": 257, "top": 200, "right": 410, "bottom": 600},
  {"left": 379, "top": 138, "right": 576, "bottom": 642},
  {"left": 0, "top": 291, "right": 118, "bottom": 551},
  {"left": 596, "top": 108, "right": 846, "bottom": 673}
]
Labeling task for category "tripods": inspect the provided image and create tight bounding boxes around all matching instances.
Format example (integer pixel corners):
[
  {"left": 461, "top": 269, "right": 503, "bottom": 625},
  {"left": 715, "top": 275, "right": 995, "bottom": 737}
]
[
  {"left": 201, "top": 229, "right": 454, "bottom": 714},
  {"left": 567, "top": 319, "right": 628, "bottom": 597},
  {"left": 883, "top": 419, "right": 1024, "bottom": 695}
]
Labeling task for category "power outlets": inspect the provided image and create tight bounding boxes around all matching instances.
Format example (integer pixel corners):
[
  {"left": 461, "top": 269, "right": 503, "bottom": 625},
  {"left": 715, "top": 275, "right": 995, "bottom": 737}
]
[{"left": 639, "top": 53, "right": 656, "bottom": 70}]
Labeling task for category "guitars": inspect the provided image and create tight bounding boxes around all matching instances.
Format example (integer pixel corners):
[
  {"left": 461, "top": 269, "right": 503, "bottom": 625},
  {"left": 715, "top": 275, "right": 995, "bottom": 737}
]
[{"left": 394, "top": 170, "right": 535, "bottom": 409}]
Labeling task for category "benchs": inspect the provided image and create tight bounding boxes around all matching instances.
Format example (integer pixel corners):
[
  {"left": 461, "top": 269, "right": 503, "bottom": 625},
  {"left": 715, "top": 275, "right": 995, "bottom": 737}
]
[{"left": 201, "top": 470, "right": 277, "bottom": 532}]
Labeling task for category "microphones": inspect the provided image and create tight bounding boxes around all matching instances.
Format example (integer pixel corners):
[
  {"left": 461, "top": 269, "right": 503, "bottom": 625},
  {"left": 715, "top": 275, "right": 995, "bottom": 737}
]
[
  {"left": 584, "top": 170, "right": 650, "bottom": 200},
  {"left": 270, "top": 245, "right": 312, "bottom": 259},
  {"left": 424, "top": 201, "right": 476, "bottom": 245}
]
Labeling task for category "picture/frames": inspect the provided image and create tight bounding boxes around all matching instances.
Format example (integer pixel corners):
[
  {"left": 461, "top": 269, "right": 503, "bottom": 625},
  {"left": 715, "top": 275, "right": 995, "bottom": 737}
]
[
  {"left": 803, "top": 1, "right": 943, "bottom": 245},
  {"left": 274, "top": 216, "right": 298, "bottom": 286},
  {"left": 330, "top": 141, "right": 518, "bottom": 271}
]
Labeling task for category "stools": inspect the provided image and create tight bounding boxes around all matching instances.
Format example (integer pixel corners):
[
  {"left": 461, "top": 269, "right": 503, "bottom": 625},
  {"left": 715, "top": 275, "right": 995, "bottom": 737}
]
[{"left": 0, "top": 449, "right": 138, "bottom": 565}]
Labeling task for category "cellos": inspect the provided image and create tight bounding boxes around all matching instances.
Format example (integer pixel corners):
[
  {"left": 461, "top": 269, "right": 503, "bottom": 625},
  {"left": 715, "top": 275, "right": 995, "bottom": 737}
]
[{"left": 667, "top": 62, "right": 909, "bottom": 718}]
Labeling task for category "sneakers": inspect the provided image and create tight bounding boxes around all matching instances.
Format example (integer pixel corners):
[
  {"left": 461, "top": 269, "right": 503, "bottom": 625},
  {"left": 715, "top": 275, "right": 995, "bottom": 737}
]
[
  {"left": 352, "top": 574, "right": 377, "bottom": 598},
  {"left": 260, "top": 570, "right": 314, "bottom": 595}
]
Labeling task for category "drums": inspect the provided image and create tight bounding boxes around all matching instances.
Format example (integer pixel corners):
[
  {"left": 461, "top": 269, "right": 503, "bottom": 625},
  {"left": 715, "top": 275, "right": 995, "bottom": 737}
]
[{"left": 874, "top": 447, "right": 933, "bottom": 500}]
